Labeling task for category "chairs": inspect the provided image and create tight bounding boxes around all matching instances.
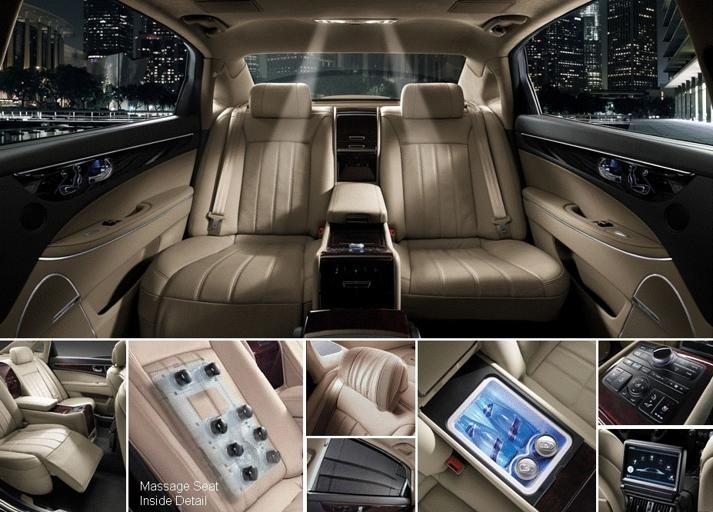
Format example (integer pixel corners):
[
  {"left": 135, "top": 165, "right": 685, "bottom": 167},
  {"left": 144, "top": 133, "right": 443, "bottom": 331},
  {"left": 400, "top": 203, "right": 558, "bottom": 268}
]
[
  {"left": 0, "top": 340, "right": 126, "bottom": 496},
  {"left": 313, "top": 346, "right": 415, "bottom": 436}
]
